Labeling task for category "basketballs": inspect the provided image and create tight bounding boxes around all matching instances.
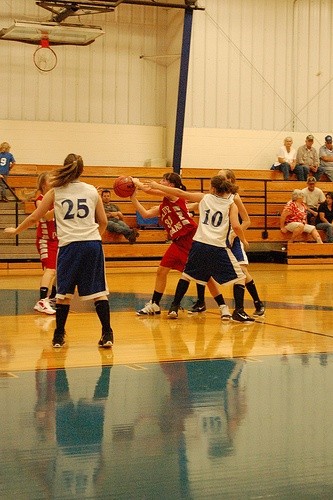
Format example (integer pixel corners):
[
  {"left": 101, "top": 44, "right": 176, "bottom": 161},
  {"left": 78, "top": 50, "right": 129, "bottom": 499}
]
[{"left": 112, "top": 175, "right": 135, "bottom": 198}]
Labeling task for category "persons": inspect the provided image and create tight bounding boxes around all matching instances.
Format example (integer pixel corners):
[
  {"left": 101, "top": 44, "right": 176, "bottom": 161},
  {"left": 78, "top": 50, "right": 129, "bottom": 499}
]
[
  {"left": 318, "top": 134, "right": 333, "bottom": 182},
  {"left": 101, "top": 189, "right": 140, "bottom": 244},
  {"left": 296, "top": 134, "right": 324, "bottom": 182},
  {"left": 314, "top": 192, "right": 333, "bottom": 242},
  {"left": 280, "top": 188, "right": 322, "bottom": 244},
  {"left": 301, "top": 176, "right": 326, "bottom": 225},
  {"left": 128, "top": 172, "right": 232, "bottom": 321},
  {"left": 142, "top": 174, "right": 256, "bottom": 325},
  {"left": 0, "top": 142, "right": 16, "bottom": 202},
  {"left": 273, "top": 136, "right": 304, "bottom": 181},
  {"left": 4, "top": 152, "right": 114, "bottom": 348},
  {"left": 30, "top": 171, "right": 103, "bottom": 315},
  {"left": 153, "top": 168, "right": 266, "bottom": 318}
]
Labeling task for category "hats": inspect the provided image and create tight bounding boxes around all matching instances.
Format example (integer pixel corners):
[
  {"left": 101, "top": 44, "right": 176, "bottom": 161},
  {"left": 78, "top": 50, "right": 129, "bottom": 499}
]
[
  {"left": 307, "top": 176, "right": 316, "bottom": 183},
  {"left": 325, "top": 135, "right": 332, "bottom": 142},
  {"left": 306, "top": 134, "right": 313, "bottom": 140}
]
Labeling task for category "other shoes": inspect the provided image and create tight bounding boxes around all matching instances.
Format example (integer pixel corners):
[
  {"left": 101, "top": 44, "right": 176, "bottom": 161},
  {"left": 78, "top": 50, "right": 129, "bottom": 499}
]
[
  {"left": 128, "top": 228, "right": 139, "bottom": 243},
  {"left": 3, "top": 197, "right": 9, "bottom": 202}
]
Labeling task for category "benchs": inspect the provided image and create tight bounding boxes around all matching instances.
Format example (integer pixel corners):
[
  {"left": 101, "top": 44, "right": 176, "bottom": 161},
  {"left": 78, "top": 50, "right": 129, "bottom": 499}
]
[{"left": 0, "top": 164, "right": 333, "bottom": 265}]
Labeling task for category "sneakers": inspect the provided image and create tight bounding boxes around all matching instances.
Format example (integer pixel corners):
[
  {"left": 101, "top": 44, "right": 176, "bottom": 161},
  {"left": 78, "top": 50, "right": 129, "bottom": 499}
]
[
  {"left": 187, "top": 300, "right": 206, "bottom": 314},
  {"left": 252, "top": 301, "right": 265, "bottom": 316},
  {"left": 167, "top": 303, "right": 183, "bottom": 319},
  {"left": 231, "top": 309, "right": 254, "bottom": 324},
  {"left": 136, "top": 300, "right": 160, "bottom": 316},
  {"left": 218, "top": 304, "right": 231, "bottom": 320},
  {"left": 53, "top": 329, "right": 66, "bottom": 348},
  {"left": 33, "top": 301, "right": 56, "bottom": 315},
  {"left": 98, "top": 329, "right": 114, "bottom": 347},
  {"left": 49, "top": 298, "right": 56, "bottom": 306}
]
